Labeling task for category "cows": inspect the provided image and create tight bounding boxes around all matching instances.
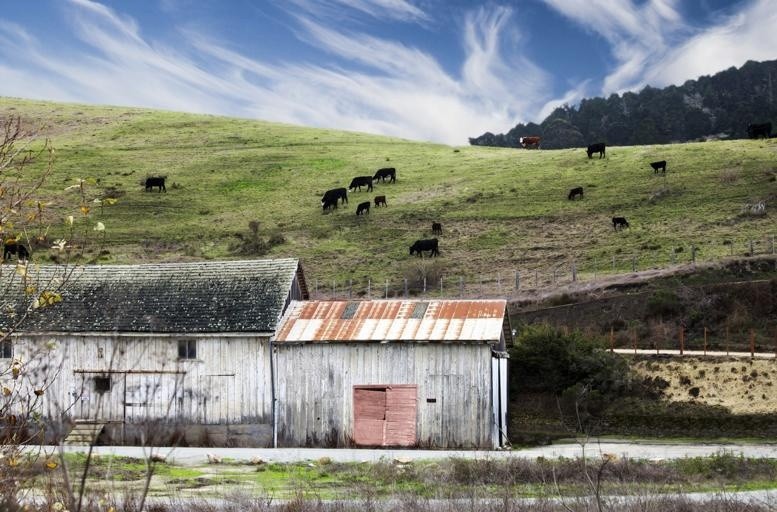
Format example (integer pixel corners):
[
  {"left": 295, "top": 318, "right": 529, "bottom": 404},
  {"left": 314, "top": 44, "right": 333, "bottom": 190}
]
[
  {"left": 409, "top": 238, "right": 442, "bottom": 258},
  {"left": 567, "top": 187, "right": 585, "bottom": 202},
  {"left": 321, "top": 187, "right": 349, "bottom": 213},
  {"left": 355, "top": 202, "right": 370, "bottom": 215},
  {"left": 373, "top": 167, "right": 397, "bottom": 184},
  {"left": 612, "top": 217, "right": 630, "bottom": 231},
  {"left": 349, "top": 175, "right": 372, "bottom": 193},
  {"left": 145, "top": 176, "right": 165, "bottom": 191},
  {"left": 518, "top": 136, "right": 540, "bottom": 151},
  {"left": 432, "top": 220, "right": 443, "bottom": 235},
  {"left": 585, "top": 143, "right": 607, "bottom": 159},
  {"left": 1, "top": 237, "right": 32, "bottom": 264},
  {"left": 375, "top": 194, "right": 386, "bottom": 207},
  {"left": 651, "top": 161, "right": 667, "bottom": 174},
  {"left": 745, "top": 121, "right": 772, "bottom": 139}
]
